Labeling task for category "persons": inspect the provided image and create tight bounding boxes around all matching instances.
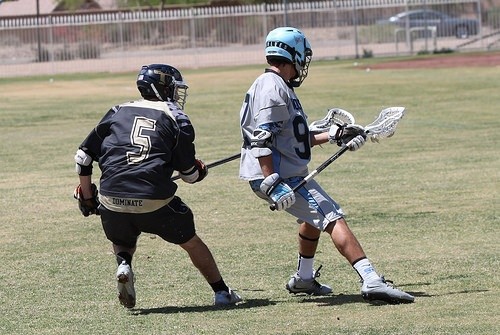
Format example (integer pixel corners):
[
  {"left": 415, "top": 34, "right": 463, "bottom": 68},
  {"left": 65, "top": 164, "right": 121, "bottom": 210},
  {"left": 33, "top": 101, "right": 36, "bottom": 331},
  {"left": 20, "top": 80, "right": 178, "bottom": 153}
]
[
  {"left": 239, "top": 27, "right": 416, "bottom": 304},
  {"left": 75, "top": 64, "right": 248, "bottom": 309}
]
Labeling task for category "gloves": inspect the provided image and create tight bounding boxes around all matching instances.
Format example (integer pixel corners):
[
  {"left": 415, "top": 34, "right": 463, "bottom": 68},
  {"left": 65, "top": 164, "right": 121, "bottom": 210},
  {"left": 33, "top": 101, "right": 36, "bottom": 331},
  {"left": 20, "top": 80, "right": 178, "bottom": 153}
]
[
  {"left": 328, "top": 124, "right": 366, "bottom": 151},
  {"left": 195, "top": 158, "right": 209, "bottom": 183},
  {"left": 74, "top": 184, "right": 100, "bottom": 217},
  {"left": 259, "top": 172, "right": 297, "bottom": 211}
]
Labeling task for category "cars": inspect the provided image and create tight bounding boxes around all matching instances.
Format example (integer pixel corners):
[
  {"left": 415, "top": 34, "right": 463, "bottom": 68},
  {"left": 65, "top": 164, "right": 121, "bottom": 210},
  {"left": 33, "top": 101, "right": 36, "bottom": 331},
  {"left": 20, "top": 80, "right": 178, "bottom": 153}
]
[{"left": 375, "top": 9, "right": 480, "bottom": 43}]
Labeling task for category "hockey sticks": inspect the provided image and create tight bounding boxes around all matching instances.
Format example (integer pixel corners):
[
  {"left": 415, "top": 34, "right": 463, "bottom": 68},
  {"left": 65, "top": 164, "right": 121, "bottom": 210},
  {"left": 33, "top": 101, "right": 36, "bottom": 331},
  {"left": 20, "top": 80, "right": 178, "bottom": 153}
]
[
  {"left": 269, "top": 106, "right": 404, "bottom": 212},
  {"left": 170, "top": 108, "right": 356, "bottom": 180}
]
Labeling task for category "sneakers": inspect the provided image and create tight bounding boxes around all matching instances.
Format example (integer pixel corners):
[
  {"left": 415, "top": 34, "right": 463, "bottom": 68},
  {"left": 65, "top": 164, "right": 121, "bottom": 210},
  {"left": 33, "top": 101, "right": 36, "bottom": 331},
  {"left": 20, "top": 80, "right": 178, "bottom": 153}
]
[
  {"left": 214, "top": 287, "right": 242, "bottom": 304},
  {"left": 116, "top": 260, "right": 138, "bottom": 308},
  {"left": 286, "top": 265, "right": 332, "bottom": 294},
  {"left": 359, "top": 275, "right": 415, "bottom": 303}
]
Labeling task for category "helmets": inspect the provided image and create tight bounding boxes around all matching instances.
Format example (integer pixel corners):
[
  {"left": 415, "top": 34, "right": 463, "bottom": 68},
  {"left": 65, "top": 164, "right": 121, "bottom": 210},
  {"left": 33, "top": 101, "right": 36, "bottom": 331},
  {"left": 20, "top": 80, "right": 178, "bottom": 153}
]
[
  {"left": 265, "top": 26, "right": 313, "bottom": 79},
  {"left": 138, "top": 64, "right": 184, "bottom": 104}
]
